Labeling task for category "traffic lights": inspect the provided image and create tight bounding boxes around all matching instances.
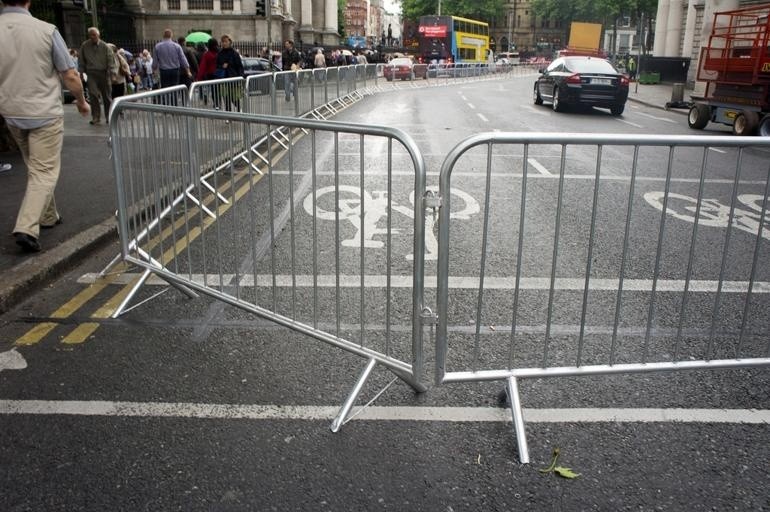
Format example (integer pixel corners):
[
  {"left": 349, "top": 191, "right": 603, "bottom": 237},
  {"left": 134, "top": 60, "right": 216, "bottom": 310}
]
[{"left": 255, "top": 0, "right": 266, "bottom": 17}]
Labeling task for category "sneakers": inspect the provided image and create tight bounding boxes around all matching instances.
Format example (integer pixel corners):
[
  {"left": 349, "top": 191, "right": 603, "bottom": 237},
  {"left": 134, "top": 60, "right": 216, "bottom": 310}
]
[
  {"left": 15, "top": 232, "right": 42, "bottom": 253},
  {"left": 39, "top": 216, "right": 63, "bottom": 230},
  {"left": 88, "top": 117, "right": 101, "bottom": 124}
]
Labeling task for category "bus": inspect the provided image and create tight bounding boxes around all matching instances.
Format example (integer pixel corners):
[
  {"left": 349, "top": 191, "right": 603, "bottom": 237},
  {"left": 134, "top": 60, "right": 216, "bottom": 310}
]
[
  {"left": 417, "top": 14, "right": 491, "bottom": 76},
  {"left": 494, "top": 52, "right": 521, "bottom": 66}
]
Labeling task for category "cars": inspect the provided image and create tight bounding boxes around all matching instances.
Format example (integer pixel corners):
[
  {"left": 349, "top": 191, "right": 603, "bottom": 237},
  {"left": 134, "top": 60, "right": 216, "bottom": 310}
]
[
  {"left": 533, "top": 55, "right": 630, "bottom": 116},
  {"left": 240, "top": 57, "right": 286, "bottom": 91},
  {"left": 383, "top": 59, "right": 417, "bottom": 81}
]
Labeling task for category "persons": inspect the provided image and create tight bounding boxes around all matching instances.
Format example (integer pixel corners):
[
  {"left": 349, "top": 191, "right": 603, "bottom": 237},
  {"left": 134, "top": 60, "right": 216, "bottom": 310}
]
[{"left": 627, "top": 56, "right": 638, "bottom": 79}]
